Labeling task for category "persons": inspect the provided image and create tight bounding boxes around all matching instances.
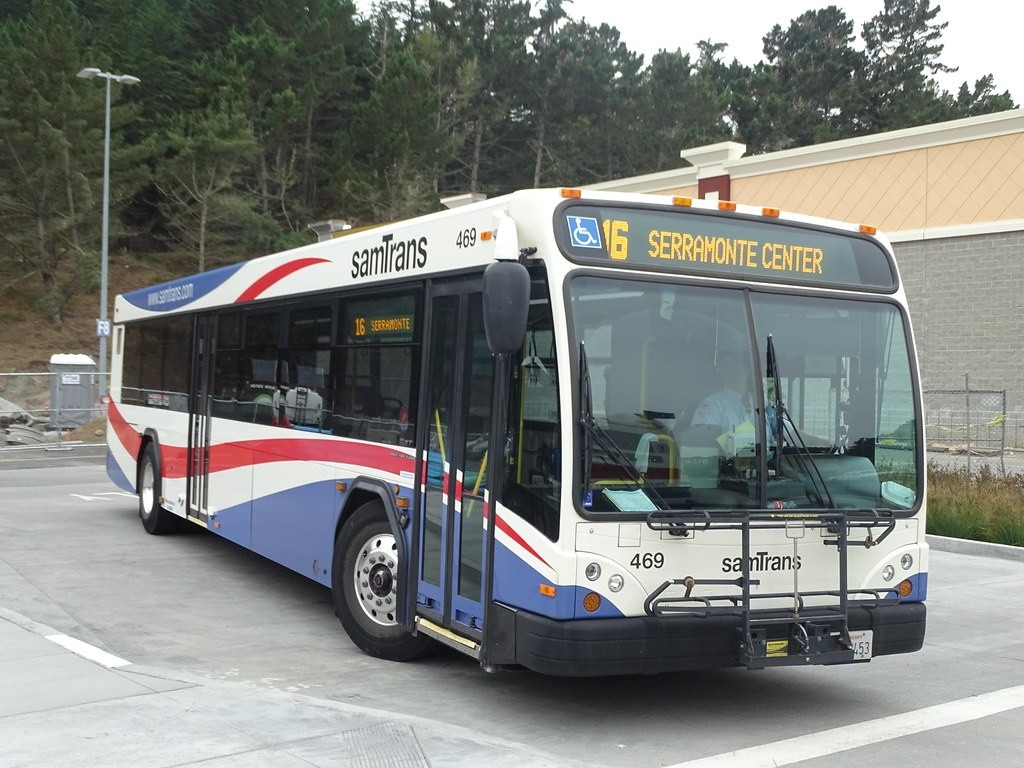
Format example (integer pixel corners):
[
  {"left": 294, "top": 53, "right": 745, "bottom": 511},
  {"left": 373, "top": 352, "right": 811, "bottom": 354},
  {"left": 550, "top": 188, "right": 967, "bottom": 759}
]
[{"left": 691, "top": 350, "right": 836, "bottom": 446}]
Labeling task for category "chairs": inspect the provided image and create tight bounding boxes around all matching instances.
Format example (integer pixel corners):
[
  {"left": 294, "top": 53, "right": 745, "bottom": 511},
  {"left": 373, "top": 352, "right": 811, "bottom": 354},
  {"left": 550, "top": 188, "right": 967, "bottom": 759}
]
[{"left": 169, "top": 345, "right": 493, "bottom": 446}]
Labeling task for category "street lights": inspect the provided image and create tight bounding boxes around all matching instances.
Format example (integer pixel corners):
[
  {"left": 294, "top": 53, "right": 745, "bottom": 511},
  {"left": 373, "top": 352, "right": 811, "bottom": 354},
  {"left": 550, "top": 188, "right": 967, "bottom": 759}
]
[{"left": 76, "top": 68, "right": 141, "bottom": 419}]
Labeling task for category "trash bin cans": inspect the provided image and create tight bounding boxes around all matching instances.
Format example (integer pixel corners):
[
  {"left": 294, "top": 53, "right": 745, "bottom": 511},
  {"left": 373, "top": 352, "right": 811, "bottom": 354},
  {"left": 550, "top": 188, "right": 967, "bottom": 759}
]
[{"left": 46, "top": 353, "right": 96, "bottom": 429}]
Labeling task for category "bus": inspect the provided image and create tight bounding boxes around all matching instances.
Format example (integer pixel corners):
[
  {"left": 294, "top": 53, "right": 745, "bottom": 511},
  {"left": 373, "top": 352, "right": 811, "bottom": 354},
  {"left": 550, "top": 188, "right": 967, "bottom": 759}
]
[{"left": 105, "top": 187, "right": 930, "bottom": 680}]
